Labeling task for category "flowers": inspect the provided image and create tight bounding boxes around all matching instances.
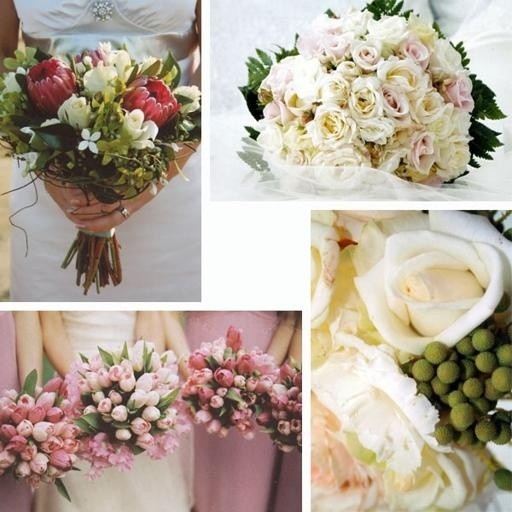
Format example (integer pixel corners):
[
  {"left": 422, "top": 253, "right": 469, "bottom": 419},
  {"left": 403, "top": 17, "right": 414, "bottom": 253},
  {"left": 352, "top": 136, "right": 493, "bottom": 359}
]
[
  {"left": 255, "top": 363, "right": 302, "bottom": 453},
  {"left": 311, "top": 210, "right": 512, "bottom": 512},
  {"left": 181, "top": 326, "right": 273, "bottom": 440},
  {"left": 0, "top": 46, "right": 202, "bottom": 297},
  {"left": 63, "top": 337, "right": 190, "bottom": 479},
  {"left": 234, "top": 0, "right": 509, "bottom": 202},
  {"left": 0, "top": 369, "right": 80, "bottom": 501}
]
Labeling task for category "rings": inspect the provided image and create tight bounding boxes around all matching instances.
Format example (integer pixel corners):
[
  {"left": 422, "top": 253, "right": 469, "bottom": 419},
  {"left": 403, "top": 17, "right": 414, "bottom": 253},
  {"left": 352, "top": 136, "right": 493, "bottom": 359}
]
[{"left": 114, "top": 207, "right": 131, "bottom": 221}]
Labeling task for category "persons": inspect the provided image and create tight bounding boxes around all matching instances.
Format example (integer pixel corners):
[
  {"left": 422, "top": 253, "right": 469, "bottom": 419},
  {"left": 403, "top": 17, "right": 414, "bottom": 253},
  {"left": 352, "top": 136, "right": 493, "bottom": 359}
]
[
  {"left": 0, "top": 0, "right": 201, "bottom": 302},
  {"left": 0, "top": 311, "right": 301, "bottom": 512}
]
